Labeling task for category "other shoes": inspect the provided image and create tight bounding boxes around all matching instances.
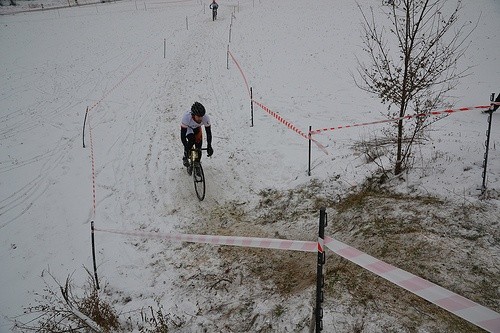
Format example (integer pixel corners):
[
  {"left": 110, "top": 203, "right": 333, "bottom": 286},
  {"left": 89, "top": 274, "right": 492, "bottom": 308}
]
[{"left": 182, "top": 157, "right": 190, "bottom": 167}]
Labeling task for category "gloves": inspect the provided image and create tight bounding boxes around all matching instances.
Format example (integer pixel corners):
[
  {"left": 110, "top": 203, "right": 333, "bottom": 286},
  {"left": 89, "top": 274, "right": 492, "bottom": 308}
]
[{"left": 206, "top": 145, "right": 213, "bottom": 157}]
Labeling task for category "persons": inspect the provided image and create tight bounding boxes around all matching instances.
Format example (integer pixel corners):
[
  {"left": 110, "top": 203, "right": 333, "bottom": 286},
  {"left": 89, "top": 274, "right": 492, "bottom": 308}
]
[
  {"left": 180, "top": 102, "right": 214, "bottom": 167},
  {"left": 209, "top": 0, "right": 219, "bottom": 15}
]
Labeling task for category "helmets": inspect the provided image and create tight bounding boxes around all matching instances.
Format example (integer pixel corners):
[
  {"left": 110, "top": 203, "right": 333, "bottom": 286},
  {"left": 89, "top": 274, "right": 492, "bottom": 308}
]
[{"left": 191, "top": 102, "right": 206, "bottom": 116}]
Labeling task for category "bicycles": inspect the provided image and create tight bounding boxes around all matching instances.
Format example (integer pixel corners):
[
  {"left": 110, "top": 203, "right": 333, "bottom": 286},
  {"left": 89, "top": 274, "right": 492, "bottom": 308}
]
[
  {"left": 210, "top": 8, "right": 218, "bottom": 21},
  {"left": 184, "top": 145, "right": 207, "bottom": 201}
]
